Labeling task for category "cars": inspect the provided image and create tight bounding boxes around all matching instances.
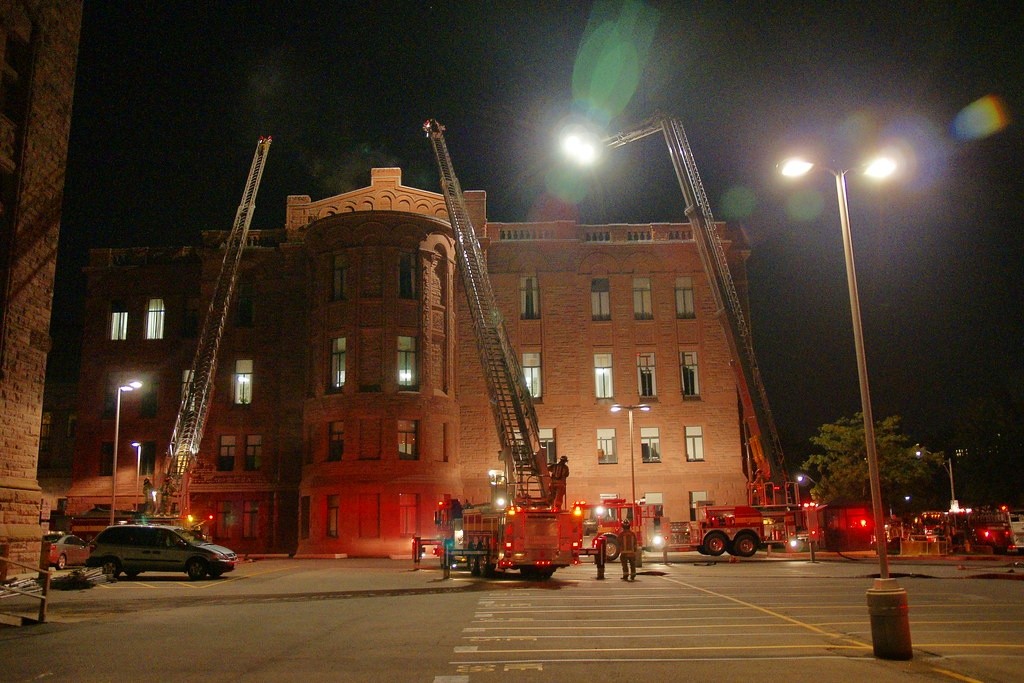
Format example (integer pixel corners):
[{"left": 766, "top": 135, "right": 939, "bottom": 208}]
[{"left": 45, "top": 530, "right": 92, "bottom": 571}]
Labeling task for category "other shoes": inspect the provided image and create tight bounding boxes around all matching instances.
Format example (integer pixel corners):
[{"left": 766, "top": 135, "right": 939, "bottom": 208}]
[
  {"left": 630, "top": 576, "right": 635, "bottom": 580},
  {"left": 621, "top": 576, "right": 628, "bottom": 580}
]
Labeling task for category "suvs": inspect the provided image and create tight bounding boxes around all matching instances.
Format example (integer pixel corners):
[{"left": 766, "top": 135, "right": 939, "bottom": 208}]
[{"left": 86, "top": 522, "right": 239, "bottom": 580}]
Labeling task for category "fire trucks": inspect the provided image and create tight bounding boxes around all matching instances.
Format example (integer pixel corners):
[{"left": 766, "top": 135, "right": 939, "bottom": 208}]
[
  {"left": 905, "top": 502, "right": 1023, "bottom": 555},
  {"left": 572, "top": 118, "right": 835, "bottom": 560},
  {"left": 49, "top": 134, "right": 274, "bottom": 546},
  {"left": 411, "top": 118, "right": 584, "bottom": 579}
]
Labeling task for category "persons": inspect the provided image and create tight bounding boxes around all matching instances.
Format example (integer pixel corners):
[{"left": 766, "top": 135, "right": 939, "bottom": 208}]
[
  {"left": 142, "top": 477, "right": 154, "bottom": 513},
  {"left": 544, "top": 456, "right": 569, "bottom": 511},
  {"left": 616, "top": 518, "right": 638, "bottom": 581},
  {"left": 158, "top": 477, "right": 175, "bottom": 517}
]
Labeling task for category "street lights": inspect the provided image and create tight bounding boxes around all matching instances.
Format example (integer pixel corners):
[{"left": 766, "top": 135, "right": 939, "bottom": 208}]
[
  {"left": 773, "top": 151, "right": 916, "bottom": 660},
  {"left": 108, "top": 379, "right": 143, "bottom": 531},
  {"left": 130, "top": 442, "right": 140, "bottom": 512},
  {"left": 609, "top": 404, "right": 652, "bottom": 548},
  {"left": 915, "top": 444, "right": 956, "bottom": 513}
]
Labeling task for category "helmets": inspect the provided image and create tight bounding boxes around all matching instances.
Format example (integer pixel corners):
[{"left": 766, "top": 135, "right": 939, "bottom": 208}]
[
  {"left": 560, "top": 456, "right": 568, "bottom": 462},
  {"left": 621, "top": 519, "right": 631, "bottom": 528}
]
